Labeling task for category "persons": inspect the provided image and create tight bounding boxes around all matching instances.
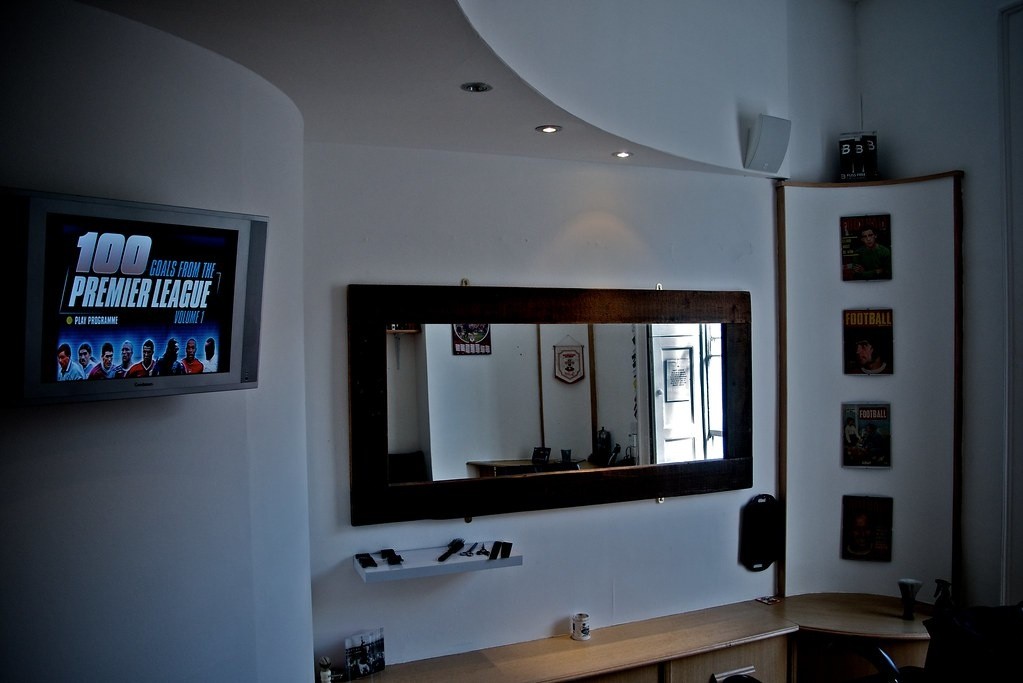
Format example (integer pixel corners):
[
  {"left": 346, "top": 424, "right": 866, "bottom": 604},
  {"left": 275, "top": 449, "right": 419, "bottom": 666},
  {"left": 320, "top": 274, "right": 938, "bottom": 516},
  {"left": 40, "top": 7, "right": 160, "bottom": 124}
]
[
  {"left": 90, "top": 343, "right": 117, "bottom": 380},
  {"left": 152, "top": 337, "right": 187, "bottom": 377},
  {"left": 58, "top": 344, "right": 86, "bottom": 380},
  {"left": 844, "top": 417, "right": 885, "bottom": 467},
  {"left": 855, "top": 335, "right": 892, "bottom": 374},
  {"left": 203, "top": 338, "right": 218, "bottom": 373},
  {"left": 78, "top": 342, "right": 98, "bottom": 380},
  {"left": 126, "top": 340, "right": 158, "bottom": 379},
  {"left": 106, "top": 341, "right": 134, "bottom": 379},
  {"left": 181, "top": 339, "right": 203, "bottom": 374},
  {"left": 859, "top": 224, "right": 892, "bottom": 272}
]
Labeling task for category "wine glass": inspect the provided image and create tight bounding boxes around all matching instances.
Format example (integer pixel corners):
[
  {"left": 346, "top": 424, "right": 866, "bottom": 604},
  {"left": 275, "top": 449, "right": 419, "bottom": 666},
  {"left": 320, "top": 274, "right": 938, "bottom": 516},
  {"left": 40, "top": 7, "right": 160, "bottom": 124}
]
[{"left": 897, "top": 578, "right": 923, "bottom": 621}]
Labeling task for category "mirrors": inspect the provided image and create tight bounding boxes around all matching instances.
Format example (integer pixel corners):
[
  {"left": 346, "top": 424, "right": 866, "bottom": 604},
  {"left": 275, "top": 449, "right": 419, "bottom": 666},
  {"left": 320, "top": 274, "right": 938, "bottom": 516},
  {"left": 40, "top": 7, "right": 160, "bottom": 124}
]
[{"left": 347, "top": 284, "right": 754, "bottom": 527}]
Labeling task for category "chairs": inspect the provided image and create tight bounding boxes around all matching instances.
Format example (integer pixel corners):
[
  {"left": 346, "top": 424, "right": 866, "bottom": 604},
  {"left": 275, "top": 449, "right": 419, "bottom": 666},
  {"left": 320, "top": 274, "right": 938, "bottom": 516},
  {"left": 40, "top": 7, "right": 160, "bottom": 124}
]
[{"left": 799, "top": 599, "right": 1023, "bottom": 682}]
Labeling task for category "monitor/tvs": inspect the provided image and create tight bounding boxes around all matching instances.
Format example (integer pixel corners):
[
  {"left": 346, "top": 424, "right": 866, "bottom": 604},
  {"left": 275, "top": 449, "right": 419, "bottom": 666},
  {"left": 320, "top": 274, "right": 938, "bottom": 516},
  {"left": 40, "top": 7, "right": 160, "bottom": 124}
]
[{"left": 0, "top": 185, "right": 270, "bottom": 402}]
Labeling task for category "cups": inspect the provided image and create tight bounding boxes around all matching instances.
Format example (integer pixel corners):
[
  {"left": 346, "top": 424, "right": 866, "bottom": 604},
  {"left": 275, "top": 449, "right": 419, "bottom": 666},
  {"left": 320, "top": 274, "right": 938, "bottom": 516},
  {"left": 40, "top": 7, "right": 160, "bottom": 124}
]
[
  {"left": 561, "top": 448, "right": 571, "bottom": 465},
  {"left": 572, "top": 613, "right": 591, "bottom": 641}
]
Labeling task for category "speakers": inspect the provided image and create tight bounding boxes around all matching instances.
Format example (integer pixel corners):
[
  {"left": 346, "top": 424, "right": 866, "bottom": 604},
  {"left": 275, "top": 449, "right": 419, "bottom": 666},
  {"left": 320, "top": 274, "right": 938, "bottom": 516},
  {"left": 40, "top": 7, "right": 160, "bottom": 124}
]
[{"left": 744, "top": 113, "right": 792, "bottom": 173}]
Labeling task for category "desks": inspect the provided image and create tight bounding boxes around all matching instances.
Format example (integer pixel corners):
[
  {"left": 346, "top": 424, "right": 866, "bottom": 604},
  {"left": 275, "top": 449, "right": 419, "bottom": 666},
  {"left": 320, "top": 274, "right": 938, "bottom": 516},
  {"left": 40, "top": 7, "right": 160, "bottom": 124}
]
[
  {"left": 334, "top": 603, "right": 809, "bottom": 683},
  {"left": 775, "top": 592, "right": 935, "bottom": 683}
]
